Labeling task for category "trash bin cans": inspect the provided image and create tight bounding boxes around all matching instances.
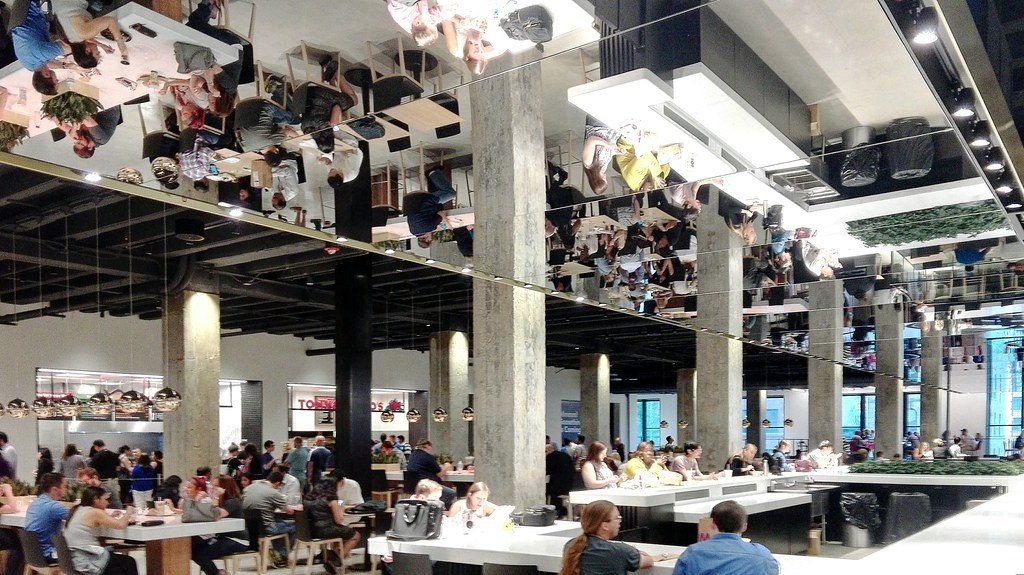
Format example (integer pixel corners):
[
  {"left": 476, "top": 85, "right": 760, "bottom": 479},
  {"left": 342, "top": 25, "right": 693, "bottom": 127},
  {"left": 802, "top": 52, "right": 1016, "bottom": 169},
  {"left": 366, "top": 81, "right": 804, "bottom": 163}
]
[
  {"left": 840, "top": 492, "right": 876, "bottom": 548},
  {"left": 841, "top": 125, "right": 877, "bottom": 188}
]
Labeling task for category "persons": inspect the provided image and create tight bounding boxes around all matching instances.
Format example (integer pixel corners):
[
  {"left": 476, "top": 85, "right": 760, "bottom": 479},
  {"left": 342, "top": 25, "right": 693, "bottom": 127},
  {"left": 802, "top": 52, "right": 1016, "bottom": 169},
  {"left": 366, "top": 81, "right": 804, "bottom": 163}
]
[
  {"left": 180, "top": 435, "right": 365, "bottom": 575},
  {"left": 11, "top": 0, "right": 363, "bottom": 210},
  {"left": 912, "top": 441, "right": 929, "bottom": 459},
  {"left": 371, "top": 433, "right": 411, "bottom": 470},
  {"left": 559, "top": 499, "right": 653, "bottom": 575},
  {"left": 613, "top": 437, "right": 624, "bottom": 463},
  {"left": 581, "top": 440, "right": 725, "bottom": 489},
  {"left": 906, "top": 432, "right": 920, "bottom": 453},
  {"left": 1015, "top": 429, "right": 1024, "bottom": 451},
  {"left": 743, "top": 244, "right": 1024, "bottom": 373},
  {"left": 670, "top": 500, "right": 781, "bottom": 575},
  {"left": 876, "top": 451, "right": 900, "bottom": 462},
  {"left": 650, "top": 435, "right": 675, "bottom": 450},
  {"left": 0, "top": 432, "right": 183, "bottom": 575},
  {"left": 381, "top": 438, "right": 498, "bottom": 575},
  {"left": 845, "top": 429, "right": 875, "bottom": 464},
  {"left": 582, "top": 116, "right": 833, "bottom": 316},
  {"left": 546, "top": 434, "right": 587, "bottom": 505},
  {"left": 404, "top": 165, "right": 586, "bottom": 293},
  {"left": 724, "top": 439, "right": 843, "bottom": 475},
  {"left": 941, "top": 428, "right": 982, "bottom": 457}
]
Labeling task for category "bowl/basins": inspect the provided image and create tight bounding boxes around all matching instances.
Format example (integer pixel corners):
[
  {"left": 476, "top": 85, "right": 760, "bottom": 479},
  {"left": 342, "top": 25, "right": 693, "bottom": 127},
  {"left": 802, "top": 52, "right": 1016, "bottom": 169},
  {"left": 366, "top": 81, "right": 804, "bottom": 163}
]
[{"left": 749, "top": 470, "right": 764, "bottom": 475}]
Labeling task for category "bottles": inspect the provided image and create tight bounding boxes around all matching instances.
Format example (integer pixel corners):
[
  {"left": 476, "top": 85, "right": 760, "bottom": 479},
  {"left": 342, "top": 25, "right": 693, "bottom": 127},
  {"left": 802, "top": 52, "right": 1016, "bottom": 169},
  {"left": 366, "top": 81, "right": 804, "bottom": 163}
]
[
  {"left": 763, "top": 460, "right": 769, "bottom": 475},
  {"left": 457, "top": 461, "right": 463, "bottom": 473}
]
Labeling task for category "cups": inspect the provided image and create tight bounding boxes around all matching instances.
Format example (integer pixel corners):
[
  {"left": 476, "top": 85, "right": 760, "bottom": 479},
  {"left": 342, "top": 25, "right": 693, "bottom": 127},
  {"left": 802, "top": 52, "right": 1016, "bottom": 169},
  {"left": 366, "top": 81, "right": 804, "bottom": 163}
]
[
  {"left": 154, "top": 501, "right": 166, "bottom": 515},
  {"left": 724, "top": 470, "right": 733, "bottom": 477},
  {"left": 149, "top": 71, "right": 159, "bottom": 89}
]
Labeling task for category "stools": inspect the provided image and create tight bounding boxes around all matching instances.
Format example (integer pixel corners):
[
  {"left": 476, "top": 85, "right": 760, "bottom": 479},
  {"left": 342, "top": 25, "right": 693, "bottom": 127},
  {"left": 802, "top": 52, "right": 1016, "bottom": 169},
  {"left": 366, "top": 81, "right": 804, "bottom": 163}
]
[
  {"left": 286, "top": 40, "right": 350, "bottom": 112},
  {"left": 318, "top": 186, "right": 335, "bottom": 229},
  {"left": 364, "top": 33, "right": 424, "bottom": 97}
]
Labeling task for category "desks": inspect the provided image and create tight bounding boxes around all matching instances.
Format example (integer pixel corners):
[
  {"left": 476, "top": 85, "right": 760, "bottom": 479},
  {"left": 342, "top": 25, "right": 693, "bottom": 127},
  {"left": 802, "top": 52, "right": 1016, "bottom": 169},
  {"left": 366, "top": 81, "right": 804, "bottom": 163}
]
[
  {"left": 0, "top": 464, "right": 856, "bottom": 575},
  {"left": 4, "top": 2, "right": 811, "bottom": 318}
]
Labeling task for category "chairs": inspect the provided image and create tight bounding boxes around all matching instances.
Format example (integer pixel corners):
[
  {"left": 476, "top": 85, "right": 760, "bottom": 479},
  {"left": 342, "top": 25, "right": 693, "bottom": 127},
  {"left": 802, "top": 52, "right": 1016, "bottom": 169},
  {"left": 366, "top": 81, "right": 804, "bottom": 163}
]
[
  {"left": 400, "top": 140, "right": 445, "bottom": 216},
  {"left": 292, "top": 509, "right": 345, "bottom": 575},
  {"left": 188, "top": 0, "right": 256, "bottom": 86},
  {"left": 422, "top": 64, "right": 463, "bottom": 140},
  {"left": 49, "top": 534, "right": 81, "bottom": 575},
  {"left": 247, "top": 507, "right": 291, "bottom": 573},
  {"left": 18, "top": 531, "right": 60, "bottom": 575},
  {"left": 221, "top": 518, "right": 261, "bottom": 575},
  {"left": 376, "top": 71, "right": 411, "bottom": 153},
  {"left": 366, "top": 161, "right": 402, "bottom": 228}
]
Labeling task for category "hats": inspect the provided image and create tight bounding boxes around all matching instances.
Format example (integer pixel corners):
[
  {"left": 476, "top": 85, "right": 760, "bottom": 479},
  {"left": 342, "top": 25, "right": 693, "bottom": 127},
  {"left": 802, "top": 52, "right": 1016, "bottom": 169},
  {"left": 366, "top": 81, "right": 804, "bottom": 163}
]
[{"left": 191, "top": 475, "right": 208, "bottom": 491}]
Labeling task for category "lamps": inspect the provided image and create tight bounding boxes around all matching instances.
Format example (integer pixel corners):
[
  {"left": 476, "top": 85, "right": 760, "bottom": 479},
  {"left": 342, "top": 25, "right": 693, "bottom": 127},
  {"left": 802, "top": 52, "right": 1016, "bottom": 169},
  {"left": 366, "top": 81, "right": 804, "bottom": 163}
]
[
  {"left": 381, "top": 285, "right": 474, "bottom": 422},
  {"left": 175, "top": 219, "right": 206, "bottom": 241},
  {"left": 0, "top": 185, "right": 181, "bottom": 416},
  {"left": 324, "top": 242, "right": 340, "bottom": 254},
  {"left": 910, "top": 7, "right": 1023, "bottom": 211}
]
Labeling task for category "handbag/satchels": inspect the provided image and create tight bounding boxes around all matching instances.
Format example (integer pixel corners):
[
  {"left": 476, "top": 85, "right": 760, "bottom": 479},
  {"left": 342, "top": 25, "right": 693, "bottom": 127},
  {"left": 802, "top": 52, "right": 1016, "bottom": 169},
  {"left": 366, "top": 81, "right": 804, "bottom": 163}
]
[
  {"left": 386, "top": 499, "right": 446, "bottom": 541},
  {"left": 347, "top": 499, "right": 388, "bottom": 516},
  {"left": 173, "top": 41, "right": 217, "bottom": 74},
  {"left": 794, "top": 226, "right": 811, "bottom": 239},
  {"left": 181, "top": 498, "right": 220, "bottom": 524},
  {"left": 347, "top": 116, "right": 386, "bottom": 140},
  {"left": 795, "top": 460, "right": 812, "bottom": 472},
  {"left": 120, "top": 467, "right": 129, "bottom": 475}
]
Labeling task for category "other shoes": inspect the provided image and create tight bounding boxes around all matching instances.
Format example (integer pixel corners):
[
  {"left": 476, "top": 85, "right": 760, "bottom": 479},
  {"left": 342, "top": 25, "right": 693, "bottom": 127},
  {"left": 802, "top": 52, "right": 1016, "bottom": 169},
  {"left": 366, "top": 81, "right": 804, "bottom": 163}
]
[
  {"left": 425, "top": 164, "right": 445, "bottom": 178},
  {"left": 201, "top": 0, "right": 223, "bottom": 19},
  {"left": 322, "top": 549, "right": 342, "bottom": 575},
  {"left": 318, "top": 53, "right": 340, "bottom": 81}
]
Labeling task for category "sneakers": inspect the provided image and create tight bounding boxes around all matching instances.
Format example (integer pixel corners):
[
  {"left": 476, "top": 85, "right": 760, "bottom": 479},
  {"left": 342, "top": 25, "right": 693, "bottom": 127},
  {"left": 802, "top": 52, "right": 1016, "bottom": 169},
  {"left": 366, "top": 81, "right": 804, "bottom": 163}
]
[
  {"left": 264, "top": 73, "right": 284, "bottom": 94},
  {"left": 268, "top": 549, "right": 292, "bottom": 569}
]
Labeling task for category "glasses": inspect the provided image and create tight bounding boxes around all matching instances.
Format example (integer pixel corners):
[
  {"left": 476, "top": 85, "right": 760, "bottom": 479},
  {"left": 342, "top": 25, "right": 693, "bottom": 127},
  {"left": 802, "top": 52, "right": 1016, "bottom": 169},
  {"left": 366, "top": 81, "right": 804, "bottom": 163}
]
[
  {"left": 605, "top": 515, "right": 622, "bottom": 523},
  {"left": 74, "top": 141, "right": 86, "bottom": 147}
]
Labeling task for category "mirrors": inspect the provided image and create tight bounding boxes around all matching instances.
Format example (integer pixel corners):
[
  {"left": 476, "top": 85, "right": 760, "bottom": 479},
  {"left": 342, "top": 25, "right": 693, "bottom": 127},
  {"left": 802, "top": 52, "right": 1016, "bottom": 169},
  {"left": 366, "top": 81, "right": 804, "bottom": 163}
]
[{"left": 0, "top": 0, "right": 1024, "bottom": 393}]
